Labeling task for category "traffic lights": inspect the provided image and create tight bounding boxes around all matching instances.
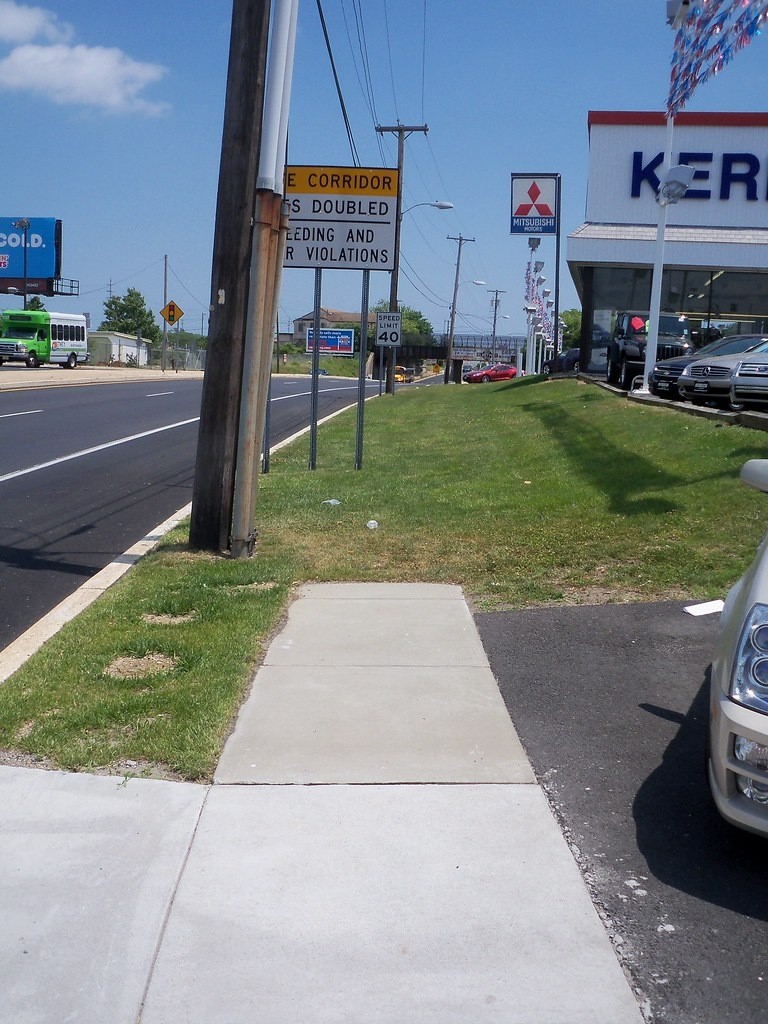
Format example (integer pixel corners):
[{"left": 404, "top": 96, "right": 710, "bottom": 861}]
[{"left": 168, "top": 305, "right": 174, "bottom": 321}]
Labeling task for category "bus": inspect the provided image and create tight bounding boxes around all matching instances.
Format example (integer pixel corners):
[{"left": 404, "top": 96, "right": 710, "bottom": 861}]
[
  {"left": 394, "top": 365, "right": 414, "bottom": 383},
  {"left": 0, "top": 310, "right": 88, "bottom": 369}
]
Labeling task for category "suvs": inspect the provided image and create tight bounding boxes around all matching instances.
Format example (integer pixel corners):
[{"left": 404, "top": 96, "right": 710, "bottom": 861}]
[{"left": 608, "top": 310, "right": 692, "bottom": 389}]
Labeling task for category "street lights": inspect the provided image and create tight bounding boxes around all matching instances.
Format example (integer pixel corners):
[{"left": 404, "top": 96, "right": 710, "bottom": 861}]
[
  {"left": 491, "top": 316, "right": 511, "bottom": 365},
  {"left": 524, "top": 237, "right": 567, "bottom": 376},
  {"left": 635, "top": 164, "right": 696, "bottom": 396},
  {"left": 386, "top": 200, "right": 453, "bottom": 390},
  {"left": 446, "top": 281, "right": 486, "bottom": 382},
  {"left": 9, "top": 218, "right": 30, "bottom": 311}
]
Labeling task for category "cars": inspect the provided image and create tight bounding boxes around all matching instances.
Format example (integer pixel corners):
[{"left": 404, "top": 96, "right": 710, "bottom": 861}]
[
  {"left": 580, "top": 348, "right": 632, "bottom": 376},
  {"left": 649, "top": 334, "right": 768, "bottom": 404},
  {"left": 679, "top": 338, "right": 768, "bottom": 406},
  {"left": 463, "top": 364, "right": 524, "bottom": 384},
  {"left": 733, "top": 348, "right": 768, "bottom": 404}
]
